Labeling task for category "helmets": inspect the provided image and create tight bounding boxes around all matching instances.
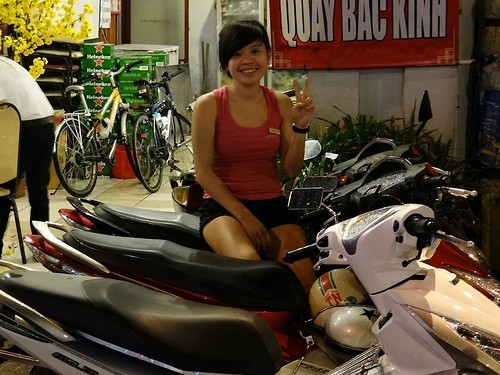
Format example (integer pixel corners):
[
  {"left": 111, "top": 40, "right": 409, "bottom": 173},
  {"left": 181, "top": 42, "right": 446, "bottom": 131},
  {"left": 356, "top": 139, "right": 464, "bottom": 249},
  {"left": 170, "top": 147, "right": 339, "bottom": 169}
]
[
  {"left": 324, "top": 305, "right": 381, "bottom": 354},
  {"left": 309, "top": 268, "right": 372, "bottom": 321}
]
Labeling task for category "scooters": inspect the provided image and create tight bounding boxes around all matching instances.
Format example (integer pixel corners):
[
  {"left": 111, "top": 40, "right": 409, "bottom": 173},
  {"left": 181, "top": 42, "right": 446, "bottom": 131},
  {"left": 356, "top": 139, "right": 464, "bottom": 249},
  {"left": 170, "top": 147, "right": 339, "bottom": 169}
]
[{"left": 0, "top": 90, "right": 500, "bottom": 375}]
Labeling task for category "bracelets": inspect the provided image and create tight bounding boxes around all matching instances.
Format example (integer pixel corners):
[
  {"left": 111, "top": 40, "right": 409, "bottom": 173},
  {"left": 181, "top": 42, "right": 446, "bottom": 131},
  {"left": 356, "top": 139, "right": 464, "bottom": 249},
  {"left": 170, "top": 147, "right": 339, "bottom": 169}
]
[{"left": 292, "top": 122, "right": 310, "bottom": 134}]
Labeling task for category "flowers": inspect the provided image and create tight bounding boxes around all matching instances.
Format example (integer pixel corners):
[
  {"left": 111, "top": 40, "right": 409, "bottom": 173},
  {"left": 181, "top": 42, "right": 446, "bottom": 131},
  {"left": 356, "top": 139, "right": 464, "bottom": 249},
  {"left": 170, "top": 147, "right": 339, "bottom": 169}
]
[{"left": 0, "top": 0, "right": 95, "bottom": 82}]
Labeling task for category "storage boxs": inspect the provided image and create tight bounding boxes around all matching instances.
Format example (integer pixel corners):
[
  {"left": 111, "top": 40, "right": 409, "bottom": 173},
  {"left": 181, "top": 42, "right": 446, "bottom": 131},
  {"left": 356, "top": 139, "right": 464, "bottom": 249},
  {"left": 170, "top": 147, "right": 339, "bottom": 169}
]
[{"left": 80, "top": 43, "right": 169, "bottom": 181}]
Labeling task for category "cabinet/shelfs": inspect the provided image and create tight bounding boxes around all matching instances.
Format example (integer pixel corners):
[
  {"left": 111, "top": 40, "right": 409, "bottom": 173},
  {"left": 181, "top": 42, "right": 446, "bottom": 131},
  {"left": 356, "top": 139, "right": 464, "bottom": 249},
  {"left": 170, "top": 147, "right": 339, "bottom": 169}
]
[{"left": 111, "top": 44, "right": 180, "bottom": 68}]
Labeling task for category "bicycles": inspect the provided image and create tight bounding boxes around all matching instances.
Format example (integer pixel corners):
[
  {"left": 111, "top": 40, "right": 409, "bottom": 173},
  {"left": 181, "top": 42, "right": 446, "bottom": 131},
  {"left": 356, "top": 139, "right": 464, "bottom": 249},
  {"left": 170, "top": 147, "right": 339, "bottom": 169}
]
[
  {"left": 131, "top": 67, "right": 199, "bottom": 192},
  {"left": 49, "top": 60, "right": 151, "bottom": 197}
]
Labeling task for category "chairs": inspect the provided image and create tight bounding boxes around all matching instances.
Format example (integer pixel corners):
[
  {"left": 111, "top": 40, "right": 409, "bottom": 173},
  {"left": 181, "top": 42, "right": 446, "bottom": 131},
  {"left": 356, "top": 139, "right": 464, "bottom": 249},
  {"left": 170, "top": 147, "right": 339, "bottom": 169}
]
[{"left": 0, "top": 101, "right": 27, "bottom": 268}]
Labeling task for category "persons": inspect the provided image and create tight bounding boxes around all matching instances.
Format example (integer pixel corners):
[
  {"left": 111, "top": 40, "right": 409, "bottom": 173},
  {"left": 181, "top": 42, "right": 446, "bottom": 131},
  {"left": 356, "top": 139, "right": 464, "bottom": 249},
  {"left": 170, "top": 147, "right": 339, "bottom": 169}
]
[
  {"left": 191, "top": 18, "right": 316, "bottom": 294},
  {"left": 0, "top": 56, "right": 56, "bottom": 260}
]
